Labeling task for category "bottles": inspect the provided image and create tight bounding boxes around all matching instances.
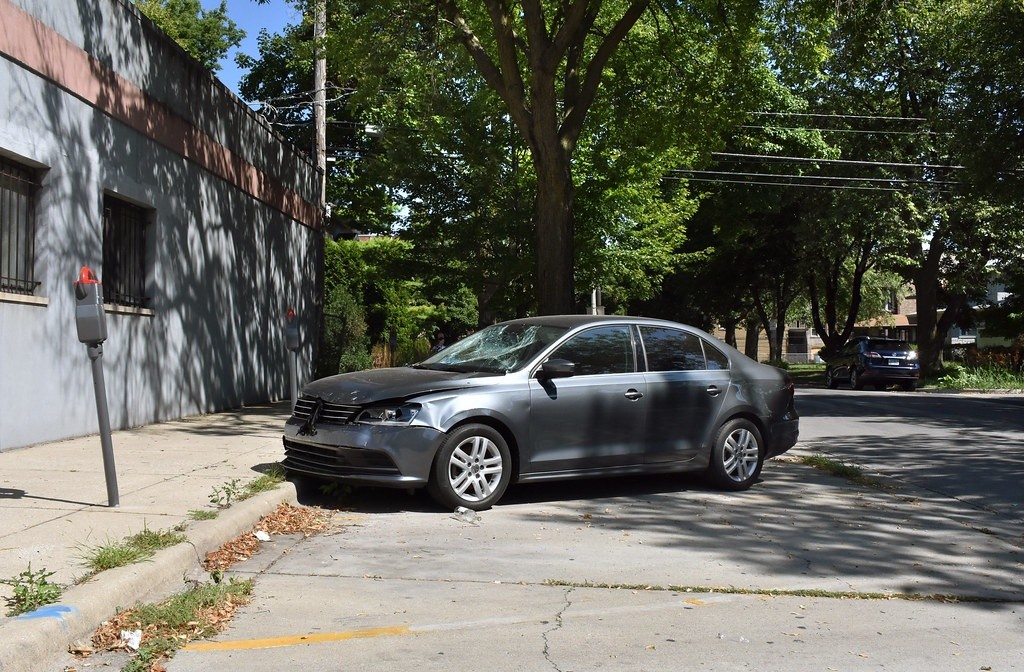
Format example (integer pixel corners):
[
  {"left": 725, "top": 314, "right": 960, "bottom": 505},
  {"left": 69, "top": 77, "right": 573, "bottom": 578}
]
[{"left": 454, "top": 505, "right": 482, "bottom": 524}]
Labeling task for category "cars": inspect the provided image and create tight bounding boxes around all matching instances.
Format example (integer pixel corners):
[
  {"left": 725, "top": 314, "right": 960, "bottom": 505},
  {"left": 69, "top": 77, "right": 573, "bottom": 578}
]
[
  {"left": 824, "top": 335, "right": 922, "bottom": 390},
  {"left": 279, "top": 313, "right": 804, "bottom": 512}
]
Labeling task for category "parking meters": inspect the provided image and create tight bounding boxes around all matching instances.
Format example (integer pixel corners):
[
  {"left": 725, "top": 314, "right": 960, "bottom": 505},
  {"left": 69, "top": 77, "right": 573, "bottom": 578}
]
[{"left": 71, "top": 265, "right": 121, "bottom": 508}]
